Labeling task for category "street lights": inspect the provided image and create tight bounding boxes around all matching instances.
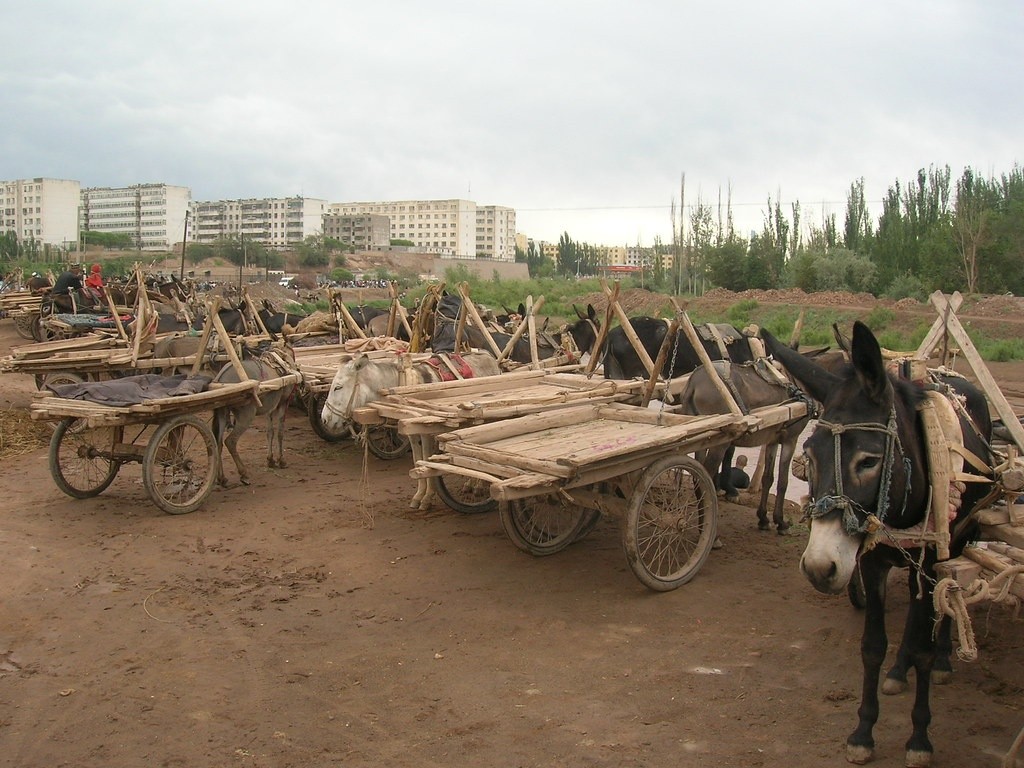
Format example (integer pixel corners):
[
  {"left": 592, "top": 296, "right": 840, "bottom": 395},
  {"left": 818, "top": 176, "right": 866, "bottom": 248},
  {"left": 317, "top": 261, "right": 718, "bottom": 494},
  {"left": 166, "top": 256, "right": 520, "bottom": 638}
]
[{"left": 574, "top": 257, "right": 582, "bottom": 277}]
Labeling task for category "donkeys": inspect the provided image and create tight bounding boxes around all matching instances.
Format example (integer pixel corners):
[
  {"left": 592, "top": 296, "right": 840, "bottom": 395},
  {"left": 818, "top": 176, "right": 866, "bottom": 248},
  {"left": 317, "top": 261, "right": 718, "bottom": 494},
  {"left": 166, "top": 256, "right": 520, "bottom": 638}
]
[
  {"left": 680, "top": 347, "right": 833, "bottom": 549},
  {"left": 760, "top": 318, "right": 991, "bottom": 768},
  {"left": 27, "top": 272, "right": 303, "bottom": 332},
  {"left": 206, "top": 331, "right": 307, "bottom": 487},
  {"left": 599, "top": 316, "right": 831, "bottom": 384},
  {"left": 346, "top": 292, "right": 599, "bottom": 365}
]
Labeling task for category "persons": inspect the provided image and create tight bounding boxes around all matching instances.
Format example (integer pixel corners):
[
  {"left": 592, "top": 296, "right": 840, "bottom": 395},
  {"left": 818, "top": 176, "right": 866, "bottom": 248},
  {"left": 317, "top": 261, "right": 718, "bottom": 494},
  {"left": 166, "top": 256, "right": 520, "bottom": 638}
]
[
  {"left": 0, "top": 273, "right": 9, "bottom": 319},
  {"left": 730, "top": 454, "right": 750, "bottom": 489},
  {"left": 86, "top": 263, "right": 105, "bottom": 289},
  {"left": 52, "top": 262, "right": 83, "bottom": 294},
  {"left": 30, "top": 272, "right": 50, "bottom": 291}
]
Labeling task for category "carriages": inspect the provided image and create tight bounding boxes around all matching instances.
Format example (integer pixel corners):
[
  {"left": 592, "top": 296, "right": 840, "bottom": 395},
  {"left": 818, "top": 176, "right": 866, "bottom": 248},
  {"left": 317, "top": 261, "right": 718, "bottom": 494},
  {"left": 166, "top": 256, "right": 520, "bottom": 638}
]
[
  {"left": 28, "top": 292, "right": 313, "bottom": 517},
  {"left": 1, "top": 258, "right": 1024, "bottom": 768}
]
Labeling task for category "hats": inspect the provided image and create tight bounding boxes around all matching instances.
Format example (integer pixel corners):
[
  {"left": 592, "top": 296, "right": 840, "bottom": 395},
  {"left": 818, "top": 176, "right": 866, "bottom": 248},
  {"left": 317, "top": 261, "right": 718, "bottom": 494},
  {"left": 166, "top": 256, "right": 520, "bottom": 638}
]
[
  {"left": 32, "top": 271, "right": 37, "bottom": 277},
  {"left": 90, "top": 264, "right": 102, "bottom": 273},
  {"left": 70, "top": 262, "right": 80, "bottom": 269}
]
